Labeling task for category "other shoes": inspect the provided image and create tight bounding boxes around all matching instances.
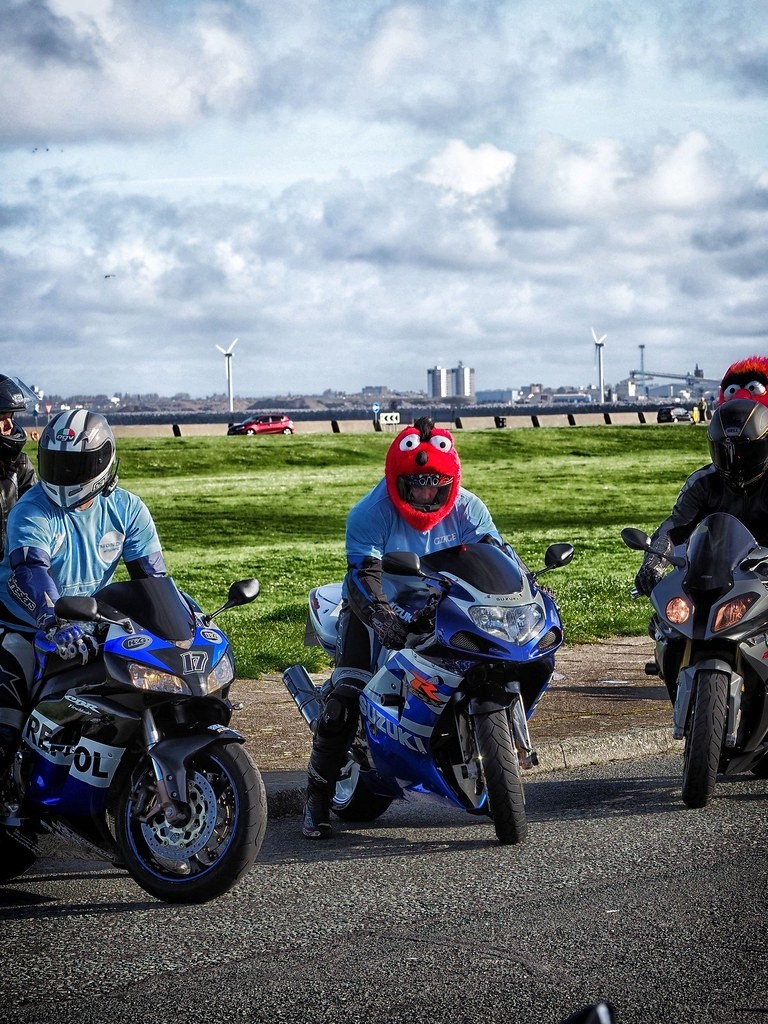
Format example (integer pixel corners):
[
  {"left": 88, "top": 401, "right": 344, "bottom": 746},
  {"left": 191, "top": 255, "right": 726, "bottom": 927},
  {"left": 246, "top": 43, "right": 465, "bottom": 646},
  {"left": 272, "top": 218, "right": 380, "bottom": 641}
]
[{"left": 0, "top": 785, "right": 24, "bottom": 818}]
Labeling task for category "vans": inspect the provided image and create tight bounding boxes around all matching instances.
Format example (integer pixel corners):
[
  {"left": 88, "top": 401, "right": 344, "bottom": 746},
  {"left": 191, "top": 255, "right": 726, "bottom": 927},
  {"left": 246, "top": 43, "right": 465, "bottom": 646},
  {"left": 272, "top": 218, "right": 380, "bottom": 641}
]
[{"left": 658, "top": 407, "right": 693, "bottom": 423}]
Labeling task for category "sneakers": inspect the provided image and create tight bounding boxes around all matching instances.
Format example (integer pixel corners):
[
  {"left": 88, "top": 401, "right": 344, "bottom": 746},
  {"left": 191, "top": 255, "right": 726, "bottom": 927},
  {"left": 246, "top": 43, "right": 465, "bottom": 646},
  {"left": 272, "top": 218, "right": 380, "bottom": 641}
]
[{"left": 301, "top": 785, "right": 333, "bottom": 840}]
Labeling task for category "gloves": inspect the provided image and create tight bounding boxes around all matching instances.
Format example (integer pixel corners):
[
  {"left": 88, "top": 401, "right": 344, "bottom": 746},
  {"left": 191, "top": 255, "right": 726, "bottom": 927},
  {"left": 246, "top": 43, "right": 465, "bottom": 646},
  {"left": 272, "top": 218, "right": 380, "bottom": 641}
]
[
  {"left": 368, "top": 610, "right": 408, "bottom": 651},
  {"left": 44, "top": 617, "right": 100, "bottom": 667},
  {"left": 634, "top": 535, "right": 673, "bottom": 597}
]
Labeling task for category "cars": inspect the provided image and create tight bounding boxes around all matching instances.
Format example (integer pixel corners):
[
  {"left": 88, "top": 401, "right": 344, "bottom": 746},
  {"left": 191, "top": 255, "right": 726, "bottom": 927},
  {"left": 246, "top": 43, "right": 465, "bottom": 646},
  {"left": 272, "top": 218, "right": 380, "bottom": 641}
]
[{"left": 227, "top": 413, "right": 295, "bottom": 436}]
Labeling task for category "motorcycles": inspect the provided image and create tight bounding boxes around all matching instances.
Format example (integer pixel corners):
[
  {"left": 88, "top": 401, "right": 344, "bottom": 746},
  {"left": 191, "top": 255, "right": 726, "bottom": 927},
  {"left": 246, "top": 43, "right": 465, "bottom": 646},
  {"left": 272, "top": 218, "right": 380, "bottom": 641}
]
[
  {"left": 621, "top": 512, "right": 768, "bottom": 809},
  {"left": 281, "top": 544, "right": 575, "bottom": 845},
  {"left": 0, "top": 576, "right": 268, "bottom": 906}
]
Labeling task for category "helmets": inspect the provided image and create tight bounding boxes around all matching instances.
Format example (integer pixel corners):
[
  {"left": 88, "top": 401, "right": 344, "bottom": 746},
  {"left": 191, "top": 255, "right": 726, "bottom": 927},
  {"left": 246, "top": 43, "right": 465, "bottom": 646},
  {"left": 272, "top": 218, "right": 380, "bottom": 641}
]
[
  {"left": 36, "top": 409, "right": 116, "bottom": 509},
  {"left": 400, "top": 473, "right": 454, "bottom": 512},
  {"left": 0, "top": 373, "right": 40, "bottom": 467},
  {"left": 707, "top": 398, "right": 768, "bottom": 485}
]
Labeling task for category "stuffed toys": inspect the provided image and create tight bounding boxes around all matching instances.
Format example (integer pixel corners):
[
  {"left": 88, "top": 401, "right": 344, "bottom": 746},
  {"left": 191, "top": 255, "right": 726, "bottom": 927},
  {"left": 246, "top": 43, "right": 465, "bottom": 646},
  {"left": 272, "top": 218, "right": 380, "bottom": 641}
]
[{"left": 719, "top": 355, "right": 768, "bottom": 408}]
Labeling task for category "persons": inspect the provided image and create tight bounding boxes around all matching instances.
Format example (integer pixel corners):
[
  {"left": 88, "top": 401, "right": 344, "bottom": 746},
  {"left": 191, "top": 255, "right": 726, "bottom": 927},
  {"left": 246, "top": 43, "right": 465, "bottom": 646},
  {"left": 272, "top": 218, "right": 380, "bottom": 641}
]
[
  {"left": 698, "top": 397, "right": 707, "bottom": 424},
  {"left": 0, "top": 409, "right": 167, "bottom": 818},
  {"left": 0, "top": 373, "right": 39, "bottom": 561},
  {"left": 635, "top": 399, "right": 768, "bottom": 641},
  {"left": 301, "top": 416, "right": 502, "bottom": 840}
]
